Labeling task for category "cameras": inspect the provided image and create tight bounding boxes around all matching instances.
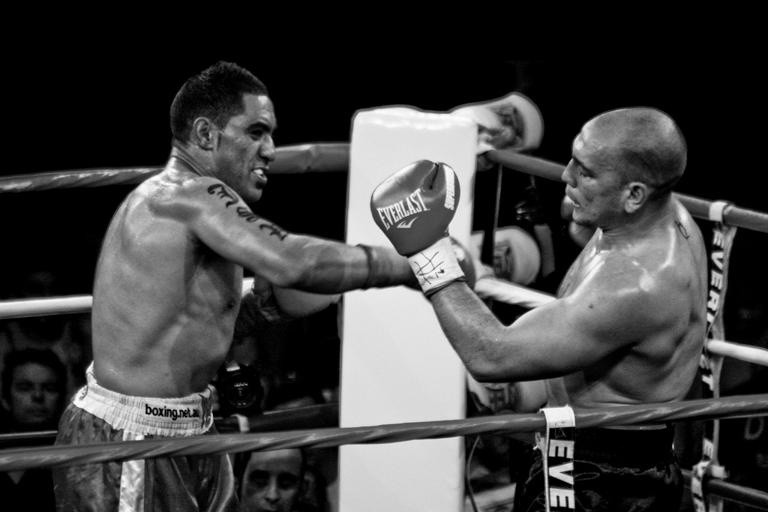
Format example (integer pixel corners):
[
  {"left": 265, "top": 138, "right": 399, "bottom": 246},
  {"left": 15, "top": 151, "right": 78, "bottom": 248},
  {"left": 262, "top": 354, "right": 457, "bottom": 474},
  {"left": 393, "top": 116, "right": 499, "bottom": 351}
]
[{"left": 222, "top": 370, "right": 263, "bottom": 413}]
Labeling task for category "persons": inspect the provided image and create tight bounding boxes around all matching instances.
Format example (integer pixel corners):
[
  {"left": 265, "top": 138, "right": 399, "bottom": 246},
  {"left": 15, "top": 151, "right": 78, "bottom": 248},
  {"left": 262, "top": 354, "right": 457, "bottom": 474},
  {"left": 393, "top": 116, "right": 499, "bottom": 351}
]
[
  {"left": 368, "top": 106, "right": 708, "bottom": 507},
  {"left": 51, "top": 62, "right": 472, "bottom": 511},
  {"left": 2, "top": 250, "right": 767, "bottom": 510}
]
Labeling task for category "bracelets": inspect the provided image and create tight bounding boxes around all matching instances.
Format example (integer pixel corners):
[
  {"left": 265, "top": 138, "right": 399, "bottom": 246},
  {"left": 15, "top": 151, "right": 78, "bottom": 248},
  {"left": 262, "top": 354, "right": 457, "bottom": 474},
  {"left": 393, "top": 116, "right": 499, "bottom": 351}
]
[
  {"left": 405, "top": 233, "right": 469, "bottom": 299},
  {"left": 351, "top": 241, "right": 377, "bottom": 293}
]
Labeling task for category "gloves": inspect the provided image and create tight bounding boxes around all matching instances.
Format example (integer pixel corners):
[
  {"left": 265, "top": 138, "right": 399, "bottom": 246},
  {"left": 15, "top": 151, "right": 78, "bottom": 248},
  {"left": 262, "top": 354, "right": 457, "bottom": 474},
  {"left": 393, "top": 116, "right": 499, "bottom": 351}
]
[
  {"left": 468, "top": 368, "right": 565, "bottom": 416},
  {"left": 365, "top": 160, "right": 475, "bottom": 300},
  {"left": 253, "top": 264, "right": 343, "bottom": 318}
]
